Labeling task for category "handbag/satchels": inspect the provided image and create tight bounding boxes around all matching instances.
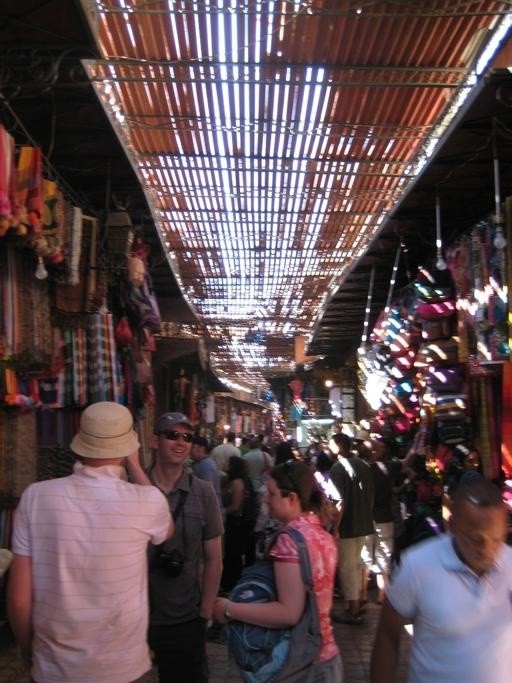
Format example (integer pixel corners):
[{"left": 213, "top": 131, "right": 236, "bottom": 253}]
[
  {"left": 115, "top": 316, "right": 155, "bottom": 404},
  {"left": 384, "top": 316, "right": 416, "bottom": 447},
  {"left": 414, "top": 267, "right": 473, "bottom": 445}
]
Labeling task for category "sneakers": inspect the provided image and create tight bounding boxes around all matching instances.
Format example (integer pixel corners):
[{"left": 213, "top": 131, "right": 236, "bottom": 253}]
[{"left": 332, "top": 611, "right": 366, "bottom": 625}]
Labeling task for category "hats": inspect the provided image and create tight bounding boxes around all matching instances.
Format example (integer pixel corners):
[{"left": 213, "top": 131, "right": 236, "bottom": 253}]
[
  {"left": 70, "top": 403, "right": 140, "bottom": 459},
  {"left": 354, "top": 429, "right": 371, "bottom": 441},
  {"left": 154, "top": 412, "right": 194, "bottom": 432}
]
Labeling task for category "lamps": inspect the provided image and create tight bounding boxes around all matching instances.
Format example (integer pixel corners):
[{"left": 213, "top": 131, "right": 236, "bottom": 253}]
[
  {"left": 435, "top": 159, "right": 506, "bottom": 271},
  {"left": 357, "top": 249, "right": 401, "bottom": 354}
]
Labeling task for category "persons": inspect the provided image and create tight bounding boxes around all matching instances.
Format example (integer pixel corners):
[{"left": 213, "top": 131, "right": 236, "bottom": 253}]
[
  {"left": 7, "top": 401, "right": 175, "bottom": 683},
  {"left": 213, "top": 462, "right": 344, "bottom": 683},
  {"left": 145, "top": 413, "right": 225, "bottom": 683},
  {"left": 169, "top": 434, "right": 480, "bottom": 645},
  {"left": 370, "top": 473, "right": 512, "bottom": 683}
]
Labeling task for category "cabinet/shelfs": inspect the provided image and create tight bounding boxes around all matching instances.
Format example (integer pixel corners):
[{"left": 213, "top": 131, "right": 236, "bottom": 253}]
[
  {"left": 214, "top": 396, "right": 274, "bottom": 435},
  {"left": 328, "top": 386, "right": 356, "bottom": 437},
  {"left": 303, "top": 398, "right": 331, "bottom": 419}
]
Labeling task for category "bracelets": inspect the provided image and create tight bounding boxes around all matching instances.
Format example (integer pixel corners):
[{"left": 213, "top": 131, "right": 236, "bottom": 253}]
[{"left": 224, "top": 602, "right": 231, "bottom": 622}]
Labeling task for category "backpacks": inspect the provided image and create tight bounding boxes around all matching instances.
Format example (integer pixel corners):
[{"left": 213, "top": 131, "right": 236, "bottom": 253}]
[{"left": 228, "top": 529, "right": 322, "bottom": 683}]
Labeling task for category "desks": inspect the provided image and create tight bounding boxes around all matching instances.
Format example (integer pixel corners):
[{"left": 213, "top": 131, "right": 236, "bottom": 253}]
[{"left": 297, "top": 419, "right": 370, "bottom": 455}]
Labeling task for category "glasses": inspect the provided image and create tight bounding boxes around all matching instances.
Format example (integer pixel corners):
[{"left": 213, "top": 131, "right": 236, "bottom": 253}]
[{"left": 159, "top": 430, "right": 193, "bottom": 441}]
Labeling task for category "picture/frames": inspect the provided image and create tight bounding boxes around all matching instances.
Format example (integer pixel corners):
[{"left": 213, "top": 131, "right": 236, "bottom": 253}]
[
  {"left": 70, "top": 206, "right": 99, "bottom": 285},
  {"left": 27, "top": 369, "right": 66, "bottom": 409}
]
